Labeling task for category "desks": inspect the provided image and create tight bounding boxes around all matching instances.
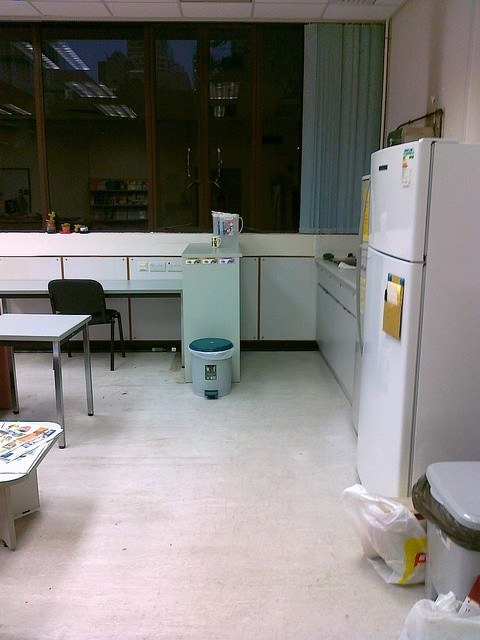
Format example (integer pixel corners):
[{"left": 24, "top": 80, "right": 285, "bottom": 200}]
[
  {"left": 0, "top": 312, "right": 100, "bottom": 449},
  {"left": 0, "top": 279, "right": 183, "bottom": 357},
  {"left": 0, "top": 420, "right": 63, "bottom": 548},
  {"left": 182, "top": 243, "right": 241, "bottom": 383}
]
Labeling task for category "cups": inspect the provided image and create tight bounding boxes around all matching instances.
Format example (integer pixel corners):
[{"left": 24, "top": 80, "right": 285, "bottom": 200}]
[{"left": 210, "top": 236, "right": 222, "bottom": 249}]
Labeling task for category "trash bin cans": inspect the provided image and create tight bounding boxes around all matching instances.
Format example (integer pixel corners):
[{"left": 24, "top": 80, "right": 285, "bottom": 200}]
[
  {"left": 424, "top": 460, "right": 480, "bottom": 601},
  {"left": 188, "top": 337, "right": 234, "bottom": 398}
]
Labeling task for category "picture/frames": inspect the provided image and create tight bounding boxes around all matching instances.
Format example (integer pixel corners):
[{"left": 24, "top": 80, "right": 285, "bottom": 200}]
[{"left": 0, "top": 167, "right": 32, "bottom": 216}]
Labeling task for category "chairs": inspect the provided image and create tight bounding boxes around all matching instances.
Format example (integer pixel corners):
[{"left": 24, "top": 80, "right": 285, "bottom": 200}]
[{"left": 47, "top": 279, "right": 126, "bottom": 371}]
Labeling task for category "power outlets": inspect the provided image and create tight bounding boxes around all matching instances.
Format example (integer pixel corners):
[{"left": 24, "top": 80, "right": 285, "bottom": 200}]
[
  {"left": 149, "top": 262, "right": 165, "bottom": 272},
  {"left": 166, "top": 262, "right": 182, "bottom": 272}
]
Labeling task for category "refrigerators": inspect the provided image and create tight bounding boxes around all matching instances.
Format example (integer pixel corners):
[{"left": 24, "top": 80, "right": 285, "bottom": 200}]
[{"left": 354, "top": 138, "right": 480, "bottom": 515}]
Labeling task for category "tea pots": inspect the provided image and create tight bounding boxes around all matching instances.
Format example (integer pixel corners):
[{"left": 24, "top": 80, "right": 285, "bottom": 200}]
[{"left": 210, "top": 211, "right": 243, "bottom": 249}]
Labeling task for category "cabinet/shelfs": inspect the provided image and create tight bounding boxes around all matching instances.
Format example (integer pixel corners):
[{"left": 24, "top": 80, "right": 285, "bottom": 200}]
[
  {"left": 315, "top": 256, "right": 356, "bottom": 398},
  {"left": 88, "top": 177, "right": 148, "bottom": 231}
]
